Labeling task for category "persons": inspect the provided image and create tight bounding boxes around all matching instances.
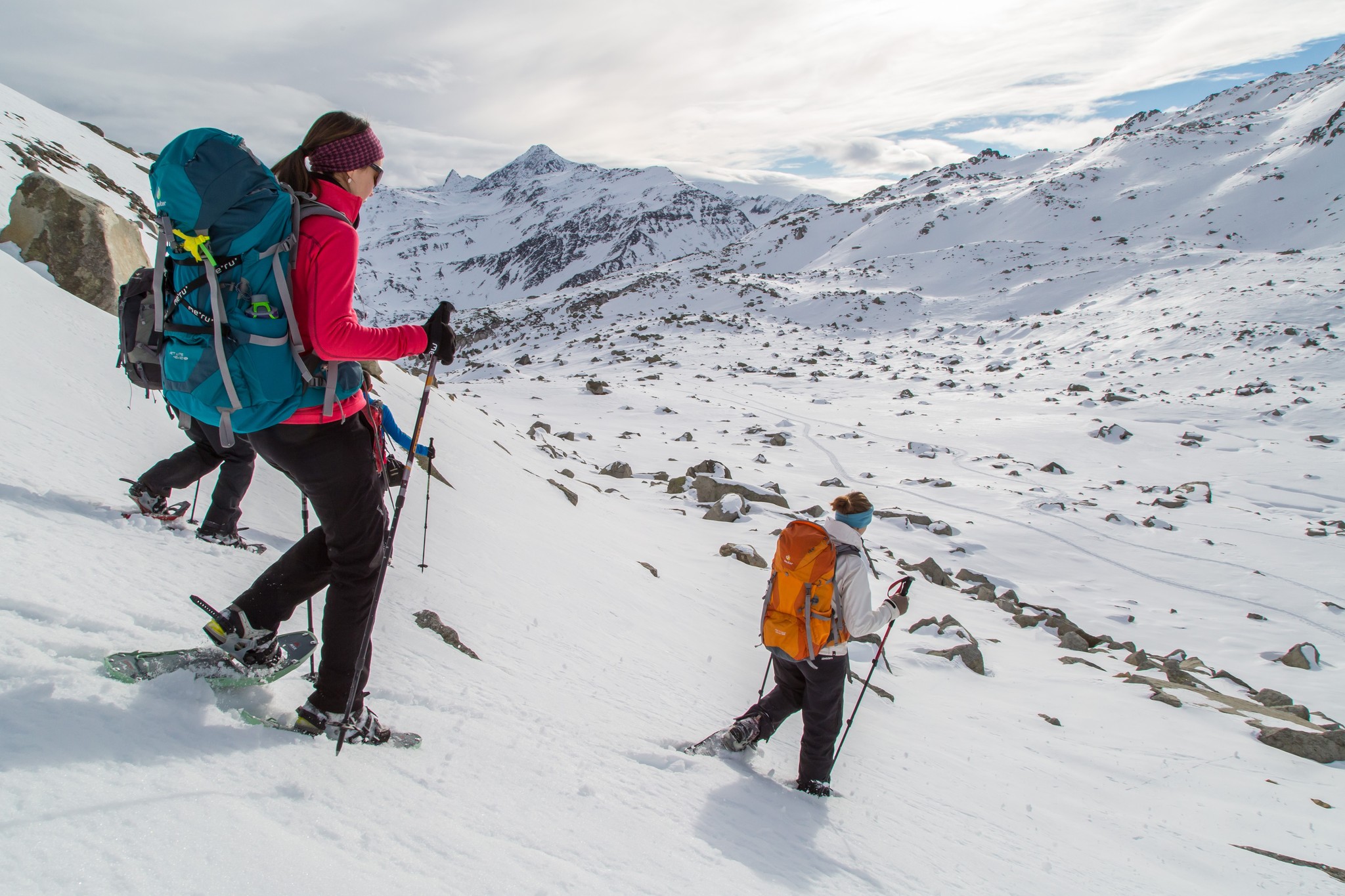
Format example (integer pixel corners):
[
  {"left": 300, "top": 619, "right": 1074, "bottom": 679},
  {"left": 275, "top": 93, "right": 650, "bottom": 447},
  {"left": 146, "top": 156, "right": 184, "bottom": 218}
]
[
  {"left": 124, "top": 390, "right": 262, "bottom": 557},
  {"left": 203, "top": 110, "right": 460, "bottom": 748},
  {"left": 725, "top": 487, "right": 909, "bottom": 798}
]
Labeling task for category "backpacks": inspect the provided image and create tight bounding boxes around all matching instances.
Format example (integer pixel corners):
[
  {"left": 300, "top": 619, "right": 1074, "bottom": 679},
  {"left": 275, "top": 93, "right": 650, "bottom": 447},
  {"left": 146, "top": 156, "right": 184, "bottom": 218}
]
[
  {"left": 761, "top": 518, "right": 861, "bottom": 663},
  {"left": 118, "top": 265, "right": 164, "bottom": 391},
  {"left": 148, "top": 126, "right": 365, "bottom": 434}
]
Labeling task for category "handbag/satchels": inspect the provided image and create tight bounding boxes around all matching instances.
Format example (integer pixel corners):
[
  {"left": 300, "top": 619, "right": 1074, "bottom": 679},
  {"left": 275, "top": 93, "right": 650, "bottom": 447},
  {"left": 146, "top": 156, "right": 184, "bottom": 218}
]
[{"left": 386, "top": 452, "right": 405, "bottom": 487}]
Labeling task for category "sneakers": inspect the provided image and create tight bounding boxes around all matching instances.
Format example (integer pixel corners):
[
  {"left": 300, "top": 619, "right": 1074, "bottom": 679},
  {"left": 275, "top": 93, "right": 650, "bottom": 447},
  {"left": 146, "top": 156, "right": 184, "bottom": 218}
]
[
  {"left": 203, "top": 604, "right": 281, "bottom": 672},
  {"left": 200, "top": 524, "right": 247, "bottom": 550},
  {"left": 132, "top": 480, "right": 171, "bottom": 515},
  {"left": 296, "top": 699, "right": 390, "bottom": 744}
]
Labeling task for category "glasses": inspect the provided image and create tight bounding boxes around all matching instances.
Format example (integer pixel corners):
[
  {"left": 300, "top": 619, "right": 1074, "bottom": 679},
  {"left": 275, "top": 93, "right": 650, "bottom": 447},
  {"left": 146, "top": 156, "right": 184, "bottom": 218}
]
[{"left": 370, "top": 162, "right": 384, "bottom": 188}]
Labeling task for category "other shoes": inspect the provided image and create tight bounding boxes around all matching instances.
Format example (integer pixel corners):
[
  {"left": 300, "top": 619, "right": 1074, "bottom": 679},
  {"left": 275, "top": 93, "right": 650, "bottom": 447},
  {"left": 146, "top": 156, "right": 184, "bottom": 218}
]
[{"left": 728, "top": 717, "right": 760, "bottom": 752}]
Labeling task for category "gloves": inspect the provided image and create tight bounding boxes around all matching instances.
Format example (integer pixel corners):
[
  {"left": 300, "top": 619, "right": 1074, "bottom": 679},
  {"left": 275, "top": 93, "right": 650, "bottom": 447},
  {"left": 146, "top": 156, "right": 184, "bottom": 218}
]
[
  {"left": 891, "top": 591, "right": 909, "bottom": 616},
  {"left": 427, "top": 446, "right": 436, "bottom": 459},
  {"left": 420, "top": 301, "right": 457, "bottom": 366}
]
